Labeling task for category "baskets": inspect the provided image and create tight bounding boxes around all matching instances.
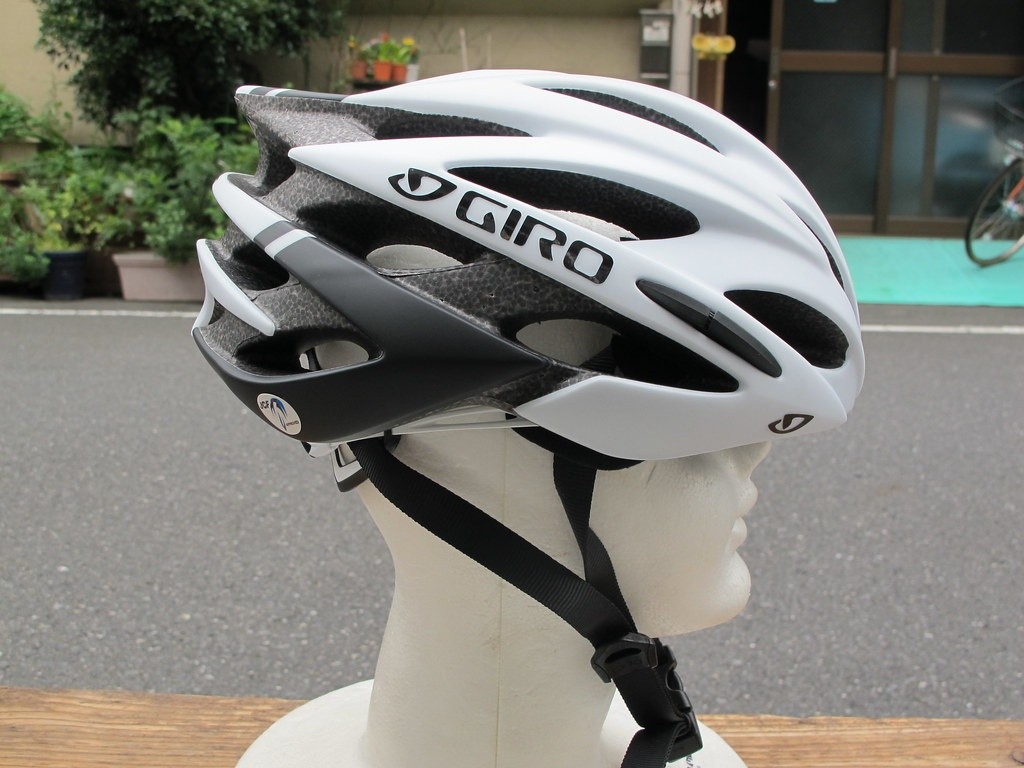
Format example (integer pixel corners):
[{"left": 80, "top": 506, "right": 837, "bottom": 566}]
[{"left": 994, "top": 76, "right": 1024, "bottom": 156}]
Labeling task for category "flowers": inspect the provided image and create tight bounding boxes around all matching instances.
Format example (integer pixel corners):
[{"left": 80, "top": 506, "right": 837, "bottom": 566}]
[{"left": 342, "top": 33, "right": 416, "bottom": 67}]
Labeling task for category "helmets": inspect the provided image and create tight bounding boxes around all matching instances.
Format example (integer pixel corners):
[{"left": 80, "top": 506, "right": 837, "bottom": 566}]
[{"left": 190, "top": 68, "right": 866, "bottom": 461}]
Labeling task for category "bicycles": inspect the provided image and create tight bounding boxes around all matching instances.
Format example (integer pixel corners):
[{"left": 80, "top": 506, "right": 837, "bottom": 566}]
[{"left": 964, "top": 76, "right": 1024, "bottom": 268}]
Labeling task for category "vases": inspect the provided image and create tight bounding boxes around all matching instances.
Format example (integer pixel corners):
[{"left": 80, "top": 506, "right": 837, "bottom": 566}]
[{"left": 348, "top": 61, "right": 421, "bottom": 86}]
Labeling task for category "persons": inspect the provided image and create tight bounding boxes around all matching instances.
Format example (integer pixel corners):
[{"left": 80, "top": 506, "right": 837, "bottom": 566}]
[{"left": 192, "top": 70, "right": 866, "bottom": 767}]
